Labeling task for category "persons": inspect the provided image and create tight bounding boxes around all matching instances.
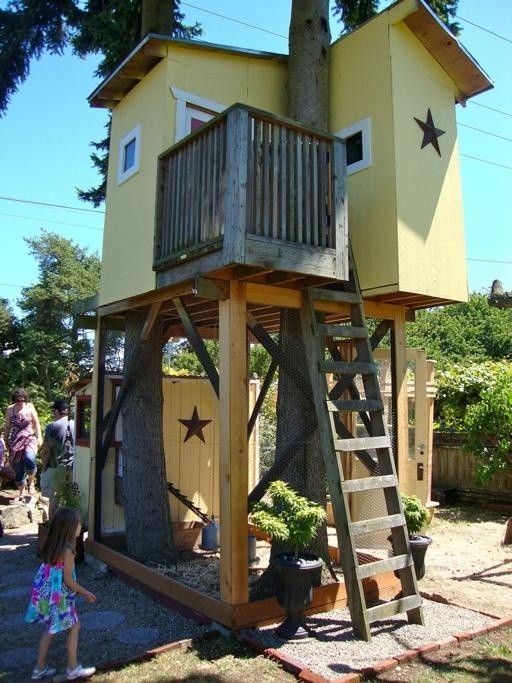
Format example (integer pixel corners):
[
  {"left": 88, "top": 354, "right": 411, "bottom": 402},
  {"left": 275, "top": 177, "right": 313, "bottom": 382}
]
[
  {"left": 0, "top": 429, "right": 16, "bottom": 539},
  {"left": 25, "top": 506, "right": 98, "bottom": 680},
  {"left": 38, "top": 400, "right": 76, "bottom": 519},
  {"left": 4, "top": 385, "right": 43, "bottom": 501}
]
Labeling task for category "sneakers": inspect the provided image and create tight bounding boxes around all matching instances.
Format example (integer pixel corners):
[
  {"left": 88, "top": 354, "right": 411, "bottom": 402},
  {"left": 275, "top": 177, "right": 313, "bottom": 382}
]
[
  {"left": 31, "top": 665, "right": 56, "bottom": 679},
  {"left": 66, "top": 663, "right": 96, "bottom": 680}
]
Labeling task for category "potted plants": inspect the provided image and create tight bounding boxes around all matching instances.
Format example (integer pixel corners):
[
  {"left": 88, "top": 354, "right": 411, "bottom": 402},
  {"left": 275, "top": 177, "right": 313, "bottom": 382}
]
[
  {"left": 249, "top": 480, "right": 328, "bottom": 639},
  {"left": 388, "top": 493, "right": 432, "bottom": 579}
]
